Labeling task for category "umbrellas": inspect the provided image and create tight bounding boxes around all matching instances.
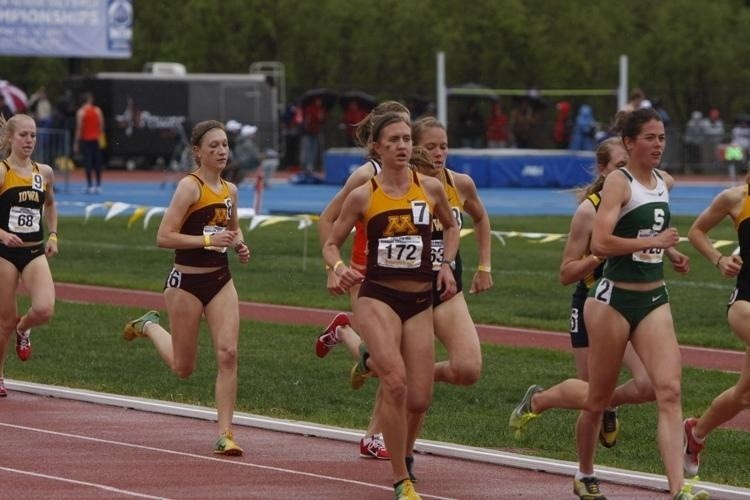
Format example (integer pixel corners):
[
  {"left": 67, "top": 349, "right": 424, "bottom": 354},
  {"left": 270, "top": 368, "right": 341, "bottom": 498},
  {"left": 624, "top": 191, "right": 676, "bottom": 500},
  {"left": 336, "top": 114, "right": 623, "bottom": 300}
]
[
  {"left": 339, "top": 90, "right": 378, "bottom": 108},
  {"left": 297, "top": 87, "right": 339, "bottom": 108}
]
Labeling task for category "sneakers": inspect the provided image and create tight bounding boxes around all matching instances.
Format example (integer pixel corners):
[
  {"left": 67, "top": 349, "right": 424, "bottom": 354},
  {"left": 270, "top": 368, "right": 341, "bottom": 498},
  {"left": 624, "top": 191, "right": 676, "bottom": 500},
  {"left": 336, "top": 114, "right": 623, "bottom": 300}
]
[
  {"left": 395, "top": 456, "right": 422, "bottom": 500},
  {"left": 683, "top": 418, "right": 705, "bottom": 476},
  {"left": 673, "top": 475, "right": 710, "bottom": 500},
  {"left": 351, "top": 343, "right": 371, "bottom": 389},
  {"left": 509, "top": 384, "right": 544, "bottom": 443},
  {"left": 211, "top": 431, "right": 244, "bottom": 456},
  {"left": 573, "top": 477, "right": 608, "bottom": 500},
  {"left": 85, "top": 185, "right": 101, "bottom": 193},
  {"left": 599, "top": 410, "right": 620, "bottom": 447},
  {"left": 16, "top": 316, "right": 31, "bottom": 361},
  {"left": 360, "top": 433, "right": 391, "bottom": 460},
  {"left": 316, "top": 313, "right": 351, "bottom": 358},
  {"left": 122, "top": 310, "right": 160, "bottom": 341},
  {"left": 0, "top": 378, "right": 7, "bottom": 396}
]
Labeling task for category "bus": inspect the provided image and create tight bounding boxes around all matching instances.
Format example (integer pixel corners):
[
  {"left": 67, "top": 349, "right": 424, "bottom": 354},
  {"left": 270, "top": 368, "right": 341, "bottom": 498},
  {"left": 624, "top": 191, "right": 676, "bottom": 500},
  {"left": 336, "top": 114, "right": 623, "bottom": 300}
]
[{"left": 443, "top": 88, "right": 617, "bottom": 149}]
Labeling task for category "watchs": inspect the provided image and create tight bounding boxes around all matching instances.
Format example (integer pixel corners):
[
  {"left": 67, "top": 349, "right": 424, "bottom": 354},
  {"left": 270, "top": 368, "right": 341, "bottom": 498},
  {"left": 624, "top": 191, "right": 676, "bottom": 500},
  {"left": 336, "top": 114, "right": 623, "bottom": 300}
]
[{"left": 441, "top": 261, "right": 455, "bottom": 269}]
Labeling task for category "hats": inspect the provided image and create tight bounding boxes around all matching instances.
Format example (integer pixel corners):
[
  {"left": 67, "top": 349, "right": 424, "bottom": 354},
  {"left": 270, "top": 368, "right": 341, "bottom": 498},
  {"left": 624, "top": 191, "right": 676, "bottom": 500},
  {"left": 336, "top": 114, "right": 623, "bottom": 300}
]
[{"left": 226, "top": 121, "right": 257, "bottom": 137}]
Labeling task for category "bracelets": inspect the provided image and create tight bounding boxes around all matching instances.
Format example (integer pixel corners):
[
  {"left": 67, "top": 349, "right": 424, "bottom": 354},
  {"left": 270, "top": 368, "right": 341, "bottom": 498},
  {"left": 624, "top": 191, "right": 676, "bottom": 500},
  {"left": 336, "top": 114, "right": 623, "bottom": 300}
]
[
  {"left": 333, "top": 261, "right": 343, "bottom": 271},
  {"left": 478, "top": 265, "right": 491, "bottom": 273},
  {"left": 204, "top": 234, "right": 212, "bottom": 247},
  {"left": 48, "top": 236, "right": 58, "bottom": 241},
  {"left": 593, "top": 255, "right": 604, "bottom": 265},
  {"left": 325, "top": 265, "right": 329, "bottom": 269},
  {"left": 48, "top": 231, "right": 57, "bottom": 235},
  {"left": 714, "top": 253, "right": 723, "bottom": 267}
]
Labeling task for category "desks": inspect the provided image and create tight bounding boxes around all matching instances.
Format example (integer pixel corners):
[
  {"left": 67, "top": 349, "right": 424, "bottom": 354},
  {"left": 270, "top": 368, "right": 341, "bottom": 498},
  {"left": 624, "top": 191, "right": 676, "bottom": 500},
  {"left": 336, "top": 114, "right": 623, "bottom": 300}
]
[{"left": 322, "top": 146, "right": 600, "bottom": 189}]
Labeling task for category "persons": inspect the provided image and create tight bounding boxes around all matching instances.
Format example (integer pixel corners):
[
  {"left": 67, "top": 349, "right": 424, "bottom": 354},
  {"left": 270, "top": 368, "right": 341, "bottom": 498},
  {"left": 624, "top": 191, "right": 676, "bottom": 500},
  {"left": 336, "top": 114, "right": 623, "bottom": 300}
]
[
  {"left": 0, "top": 94, "right": 12, "bottom": 121},
  {"left": 556, "top": 86, "right": 750, "bottom": 174},
  {"left": 573, "top": 108, "right": 710, "bottom": 500},
  {"left": 36, "top": 88, "right": 52, "bottom": 163},
  {"left": 315, "top": 111, "right": 459, "bottom": 500},
  {"left": 351, "top": 117, "right": 493, "bottom": 388},
  {"left": 0, "top": 113, "right": 59, "bottom": 397},
  {"left": 116, "top": 95, "right": 139, "bottom": 171},
  {"left": 425, "top": 98, "right": 535, "bottom": 150},
  {"left": 71, "top": 91, "right": 108, "bottom": 191},
  {"left": 123, "top": 120, "right": 251, "bottom": 457},
  {"left": 316, "top": 102, "right": 410, "bottom": 460},
  {"left": 281, "top": 99, "right": 369, "bottom": 177},
  {"left": 508, "top": 136, "right": 657, "bottom": 500},
  {"left": 221, "top": 119, "right": 263, "bottom": 185},
  {"left": 682, "top": 172, "right": 750, "bottom": 478}
]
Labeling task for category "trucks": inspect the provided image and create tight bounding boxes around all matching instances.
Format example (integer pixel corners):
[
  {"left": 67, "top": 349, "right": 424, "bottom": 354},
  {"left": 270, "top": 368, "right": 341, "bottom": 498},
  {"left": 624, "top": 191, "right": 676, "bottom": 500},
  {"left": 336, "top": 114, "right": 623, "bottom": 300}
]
[{"left": 68, "top": 72, "right": 282, "bottom": 179}]
